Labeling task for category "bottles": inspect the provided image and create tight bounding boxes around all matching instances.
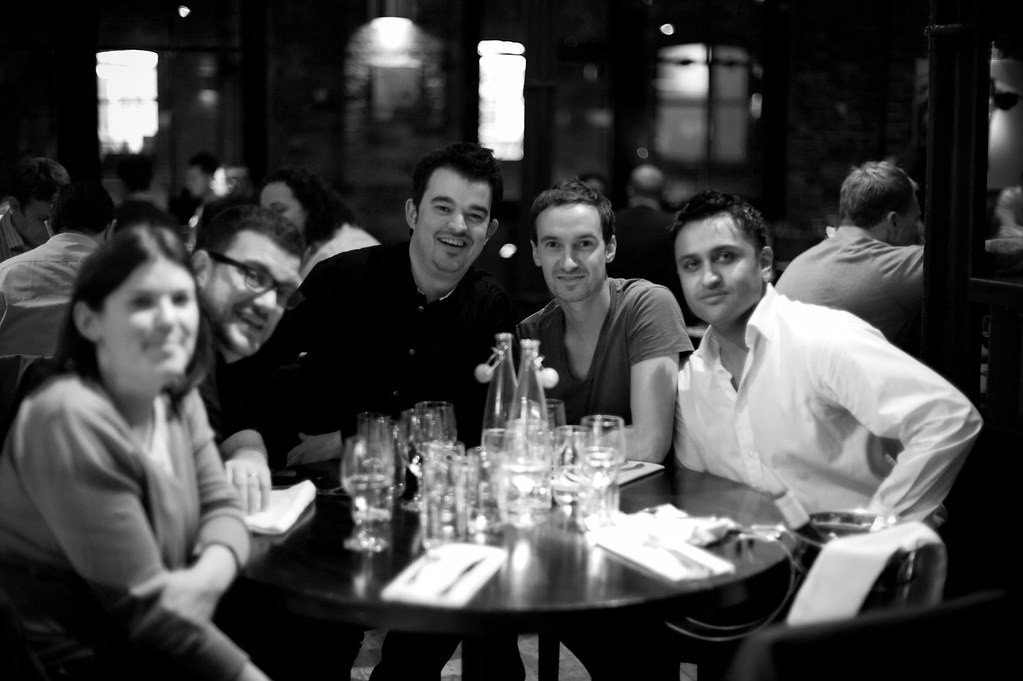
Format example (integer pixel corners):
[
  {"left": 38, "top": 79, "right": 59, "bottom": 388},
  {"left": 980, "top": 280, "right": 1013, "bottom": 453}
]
[
  {"left": 503, "top": 339, "right": 548, "bottom": 431},
  {"left": 482, "top": 333, "right": 518, "bottom": 429}
]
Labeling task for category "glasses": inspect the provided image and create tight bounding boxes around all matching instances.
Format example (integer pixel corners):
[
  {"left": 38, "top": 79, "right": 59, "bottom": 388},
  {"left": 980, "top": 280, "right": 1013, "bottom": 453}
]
[{"left": 205, "top": 248, "right": 305, "bottom": 312}]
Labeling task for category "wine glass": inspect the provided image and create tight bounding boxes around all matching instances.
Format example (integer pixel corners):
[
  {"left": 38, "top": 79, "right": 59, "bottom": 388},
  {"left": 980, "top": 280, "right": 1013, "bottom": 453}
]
[{"left": 344, "top": 402, "right": 627, "bottom": 552}]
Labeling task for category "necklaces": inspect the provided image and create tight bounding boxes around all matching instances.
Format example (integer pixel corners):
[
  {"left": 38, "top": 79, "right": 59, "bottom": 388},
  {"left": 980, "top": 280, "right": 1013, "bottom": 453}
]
[{"left": 439, "top": 286, "right": 457, "bottom": 301}]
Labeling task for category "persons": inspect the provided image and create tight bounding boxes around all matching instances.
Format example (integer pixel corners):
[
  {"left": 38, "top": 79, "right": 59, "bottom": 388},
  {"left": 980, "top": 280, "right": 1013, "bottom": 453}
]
[{"left": 0, "top": 142, "right": 1023, "bottom": 681}]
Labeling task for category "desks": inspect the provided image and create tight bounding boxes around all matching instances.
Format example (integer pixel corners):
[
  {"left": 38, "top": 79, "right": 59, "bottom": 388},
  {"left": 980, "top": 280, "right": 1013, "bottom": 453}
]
[{"left": 229, "top": 456, "right": 799, "bottom": 681}]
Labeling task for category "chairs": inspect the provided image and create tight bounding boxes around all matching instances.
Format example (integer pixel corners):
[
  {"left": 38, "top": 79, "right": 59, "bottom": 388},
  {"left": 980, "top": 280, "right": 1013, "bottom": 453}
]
[
  {"left": 0, "top": 531, "right": 174, "bottom": 681},
  {"left": 732, "top": 404, "right": 1023, "bottom": 681}
]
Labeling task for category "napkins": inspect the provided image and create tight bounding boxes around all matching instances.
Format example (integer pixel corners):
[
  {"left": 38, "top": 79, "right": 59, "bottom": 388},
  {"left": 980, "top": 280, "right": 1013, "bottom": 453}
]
[
  {"left": 377, "top": 541, "right": 510, "bottom": 611},
  {"left": 244, "top": 478, "right": 315, "bottom": 537},
  {"left": 592, "top": 527, "right": 735, "bottom": 584}
]
